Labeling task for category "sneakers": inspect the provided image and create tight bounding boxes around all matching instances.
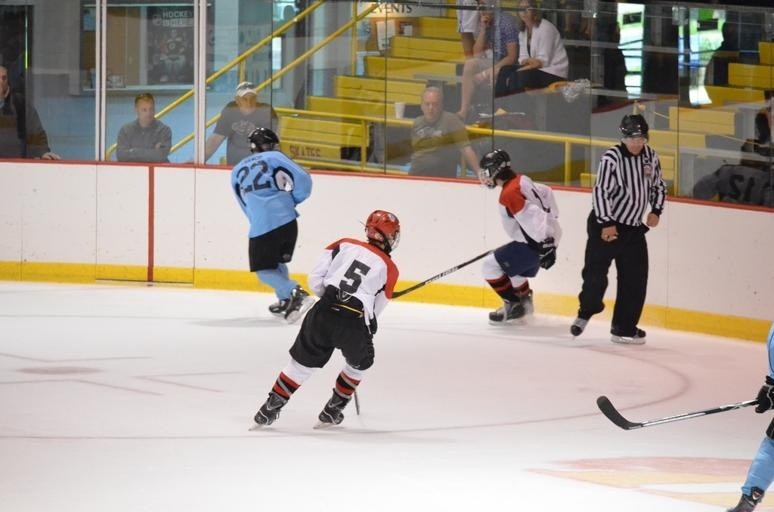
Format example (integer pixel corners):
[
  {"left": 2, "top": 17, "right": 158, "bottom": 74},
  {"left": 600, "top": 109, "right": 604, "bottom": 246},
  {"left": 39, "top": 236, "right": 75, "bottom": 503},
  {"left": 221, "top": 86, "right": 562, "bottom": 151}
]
[
  {"left": 610, "top": 324, "right": 646, "bottom": 338},
  {"left": 570, "top": 303, "right": 604, "bottom": 336}
]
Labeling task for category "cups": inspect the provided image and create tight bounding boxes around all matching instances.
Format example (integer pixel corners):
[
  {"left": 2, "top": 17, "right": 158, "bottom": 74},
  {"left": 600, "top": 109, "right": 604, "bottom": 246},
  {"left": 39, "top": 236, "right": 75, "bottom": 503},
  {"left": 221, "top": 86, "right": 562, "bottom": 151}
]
[{"left": 394, "top": 102, "right": 405, "bottom": 119}]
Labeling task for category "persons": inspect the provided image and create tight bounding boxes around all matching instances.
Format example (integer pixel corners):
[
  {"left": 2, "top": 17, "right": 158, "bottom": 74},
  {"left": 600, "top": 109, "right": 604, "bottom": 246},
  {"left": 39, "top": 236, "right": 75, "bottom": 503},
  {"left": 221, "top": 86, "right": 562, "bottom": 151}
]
[
  {"left": 115, "top": 91, "right": 172, "bottom": 163},
  {"left": 726, "top": 324, "right": 774, "bottom": 512},
  {"left": 406, "top": 83, "right": 481, "bottom": 181},
  {"left": 691, "top": 139, "right": 774, "bottom": 206},
  {"left": 570, "top": 114, "right": 666, "bottom": 339},
  {"left": 252, "top": 211, "right": 401, "bottom": 425},
  {"left": 478, "top": 146, "right": 562, "bottom": 322},
  {"left": 457, "top": 1, "right": 626, "bottom": 122},
  {"left": 229, "top": 126, "right": 313, "bottom": 321},
  {"left": 704, "top": 18, "right": 760, "bottom": 86},
  {"left": 192, "top": 82, "right": 280, "bottom": 166},
  {"left": 752, "top": 91, "right": 774, "bottom": 139},
  {"left": 0, "top": 64, "right": 60, "bottom": 160}
]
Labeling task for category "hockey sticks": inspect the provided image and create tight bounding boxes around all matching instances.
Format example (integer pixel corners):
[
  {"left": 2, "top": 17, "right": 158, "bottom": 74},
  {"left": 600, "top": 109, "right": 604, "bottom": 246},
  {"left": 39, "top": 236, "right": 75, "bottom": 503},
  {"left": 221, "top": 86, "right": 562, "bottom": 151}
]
[{"left": 595, "top": 395, "right": 765, "bottom": 431}]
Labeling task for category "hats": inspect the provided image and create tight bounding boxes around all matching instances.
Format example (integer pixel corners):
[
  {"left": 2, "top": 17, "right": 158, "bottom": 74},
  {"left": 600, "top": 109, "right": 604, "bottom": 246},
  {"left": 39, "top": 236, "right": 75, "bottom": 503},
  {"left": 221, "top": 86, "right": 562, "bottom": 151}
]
[{"left": 236, "top": 81, "right": 258, "bottom": 98}]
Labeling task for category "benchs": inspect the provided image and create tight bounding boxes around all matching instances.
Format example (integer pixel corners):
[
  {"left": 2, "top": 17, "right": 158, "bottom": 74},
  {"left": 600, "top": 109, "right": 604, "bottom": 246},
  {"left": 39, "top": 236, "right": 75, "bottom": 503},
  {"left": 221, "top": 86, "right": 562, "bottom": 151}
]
[
  {"left": 577, "top": 37, "right": 773, "bottom": 203},
  {"left": 266, "top": 0, "right": 541, "bottom": 166}
]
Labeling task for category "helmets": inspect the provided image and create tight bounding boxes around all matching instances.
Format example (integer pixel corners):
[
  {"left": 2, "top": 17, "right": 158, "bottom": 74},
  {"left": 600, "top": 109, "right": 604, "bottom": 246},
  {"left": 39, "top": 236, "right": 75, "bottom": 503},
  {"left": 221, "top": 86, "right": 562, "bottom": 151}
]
[
  {"left": 246, "top": 126, "right": 279, "bottom": 152},
  {"left": 617, "top": 114, "right": 649, "bottom": 140},
  {"left": 364, "top": 209, "right": 400, "bottom": 251},
  {"left": 477, "top": 149, "right": 511, "bottom": 189},
  {"left": 739, "top": 140, "right": 773, "bottom": 170}
]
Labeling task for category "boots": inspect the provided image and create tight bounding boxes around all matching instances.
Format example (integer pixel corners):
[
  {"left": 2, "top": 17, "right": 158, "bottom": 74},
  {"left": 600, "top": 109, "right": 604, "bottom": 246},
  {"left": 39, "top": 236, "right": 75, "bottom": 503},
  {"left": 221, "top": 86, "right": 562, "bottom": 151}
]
[
  {"left": 254, "top": 392, "right": 288, "bottom": 426},
  {"left": 318, "top": 388, "right": 352, "bottom": 425},
  {"left": 268, "top": 298, "right": 290, "bottom": 313},
  {"left": 519, "top": 290, "right": 533, "bottom": 308},
  {"left": 284, "top": 285, "right": 309, "bottom": 318},
  {"left": 725, "top": 486, "right": 763, "bottom": 512},
  {"left": 489, "top": 297, "right": 525, "bottom": 321}
]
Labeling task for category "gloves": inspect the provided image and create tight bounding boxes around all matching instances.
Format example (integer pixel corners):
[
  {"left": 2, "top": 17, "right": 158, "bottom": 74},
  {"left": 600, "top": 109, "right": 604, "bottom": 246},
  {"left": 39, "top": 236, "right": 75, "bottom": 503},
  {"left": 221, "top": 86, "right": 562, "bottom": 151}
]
[
  {"left": 539, "top": 236, "right": 556, "bottom": 269},
  {"left": 754, "top": 375, "right": 774, "bottom": 414}
]
